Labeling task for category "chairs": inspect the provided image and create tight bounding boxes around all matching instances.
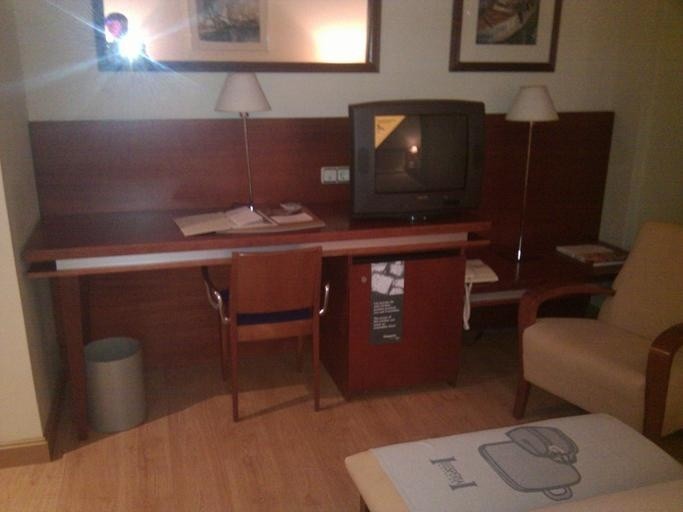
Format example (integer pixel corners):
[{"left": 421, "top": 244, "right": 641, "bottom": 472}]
[
  {"left": 201, "top": 244, "right": 329, "bottom": 421},
  {"left": 512, "top": 221, "right": 683, "bottom": 447}
]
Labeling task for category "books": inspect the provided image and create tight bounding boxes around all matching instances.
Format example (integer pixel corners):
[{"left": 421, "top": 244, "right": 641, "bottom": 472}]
[
  {"left": 225, "top": 205, "right": 263, "bottom": 228},
  {"left": 173, "top": 207, "right": 278, "bottom": 238},
  {"left": 556, "top": 244, "right": 629, "bottom": 267}
]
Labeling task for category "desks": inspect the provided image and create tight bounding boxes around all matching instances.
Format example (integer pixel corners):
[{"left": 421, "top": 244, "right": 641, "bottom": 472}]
[
  {"left": 464, "top": 255, "right": 623, "bottom": 307},
  {"left": 21, "top": 203, "right": 490, "bottom": 442}
]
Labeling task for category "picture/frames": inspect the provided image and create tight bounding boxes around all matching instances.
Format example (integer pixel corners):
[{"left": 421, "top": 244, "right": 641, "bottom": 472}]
[
  {"left": 188, "top": 0, "right": 267, "bottom": 51},
  {"left": 448, "top": 0, "right": 563, "bottom": 72}
]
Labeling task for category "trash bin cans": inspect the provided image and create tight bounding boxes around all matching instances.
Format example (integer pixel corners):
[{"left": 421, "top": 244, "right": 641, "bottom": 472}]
[{"left": 85, "top": 337, "right": 146, "bottom": 432}]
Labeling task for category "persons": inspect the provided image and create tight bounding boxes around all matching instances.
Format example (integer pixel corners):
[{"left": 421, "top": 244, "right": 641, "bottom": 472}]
[{"left": 103, "top": 13, "right": 150, "bottom": 59}]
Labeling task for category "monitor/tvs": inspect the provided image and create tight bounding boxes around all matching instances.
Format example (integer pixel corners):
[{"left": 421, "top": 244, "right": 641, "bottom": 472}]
[{"left": 348, "top": 99, "right": 484, "bottom": 224}]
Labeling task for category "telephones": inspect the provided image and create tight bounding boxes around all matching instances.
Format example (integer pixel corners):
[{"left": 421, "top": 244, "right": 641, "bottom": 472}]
[{"left": 465, "top": 260, "right": 498, "bottom": 282}]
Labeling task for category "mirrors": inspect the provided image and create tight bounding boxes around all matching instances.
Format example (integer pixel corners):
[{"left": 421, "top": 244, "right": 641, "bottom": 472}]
[{"left": 93, "top": 0, "right": 380, "bottom": 73}]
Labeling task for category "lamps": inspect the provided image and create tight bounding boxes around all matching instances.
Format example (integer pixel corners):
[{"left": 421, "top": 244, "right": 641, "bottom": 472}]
[
  {"left": 216, "top": 72, "right": 270, "bottom": 212},
  {"left": 506, "top": 86, "right": 559, "bottom": 278}
]
